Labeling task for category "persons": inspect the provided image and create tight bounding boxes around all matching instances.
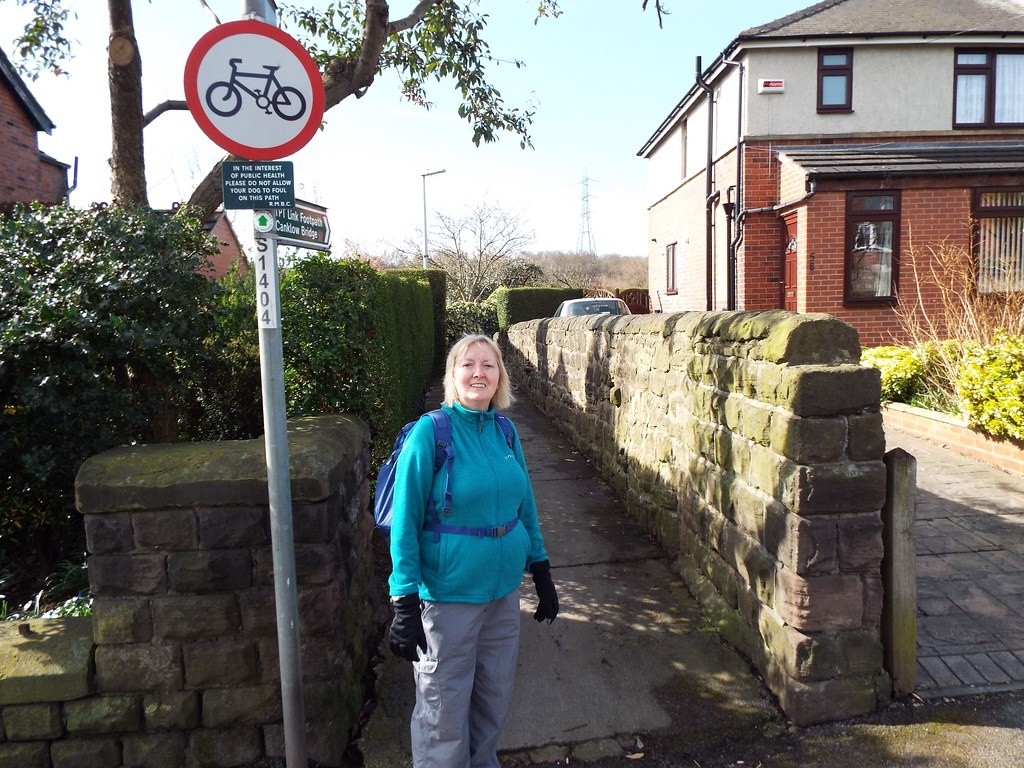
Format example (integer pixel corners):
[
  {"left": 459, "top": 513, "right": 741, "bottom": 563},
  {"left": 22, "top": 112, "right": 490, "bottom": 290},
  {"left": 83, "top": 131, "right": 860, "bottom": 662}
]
[{"left": 388, "top": 335, "right": 559, "bottom": 768}]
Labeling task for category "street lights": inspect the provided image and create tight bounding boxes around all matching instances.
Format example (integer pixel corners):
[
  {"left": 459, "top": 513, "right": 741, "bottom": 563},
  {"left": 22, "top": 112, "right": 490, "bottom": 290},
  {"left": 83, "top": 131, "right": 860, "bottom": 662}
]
[{"left": 422, "top": 166, "right": 446, "bottom": 271}]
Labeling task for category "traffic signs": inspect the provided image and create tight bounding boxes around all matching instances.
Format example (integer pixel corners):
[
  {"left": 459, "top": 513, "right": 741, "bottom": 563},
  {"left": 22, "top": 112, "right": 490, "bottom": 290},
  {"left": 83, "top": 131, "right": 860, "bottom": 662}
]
[{"left": 276, "top": 197, "right": 333, "bottom": 252}]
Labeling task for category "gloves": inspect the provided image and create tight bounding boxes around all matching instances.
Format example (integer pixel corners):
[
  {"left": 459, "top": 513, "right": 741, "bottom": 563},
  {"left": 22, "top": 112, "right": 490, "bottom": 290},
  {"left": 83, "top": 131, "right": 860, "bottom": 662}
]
[
  {"left": 529, "top": 560, "right": 559, "bottom": 625},
  {"left": 389, "top": 592, "right": 428, "bottom": 663}
]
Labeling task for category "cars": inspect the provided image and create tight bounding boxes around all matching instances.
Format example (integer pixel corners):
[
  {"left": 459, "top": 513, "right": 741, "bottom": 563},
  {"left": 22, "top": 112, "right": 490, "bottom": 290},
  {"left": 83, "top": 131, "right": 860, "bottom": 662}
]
[{"left": 552, "top": 296, "right": 632, "bottom": 317}]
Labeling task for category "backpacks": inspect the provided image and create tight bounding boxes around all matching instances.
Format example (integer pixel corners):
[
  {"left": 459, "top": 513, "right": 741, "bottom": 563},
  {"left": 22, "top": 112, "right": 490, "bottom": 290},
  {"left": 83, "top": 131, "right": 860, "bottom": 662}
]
[{"left": 373, "top": 410, "right": 520, "bottom": 542}]
